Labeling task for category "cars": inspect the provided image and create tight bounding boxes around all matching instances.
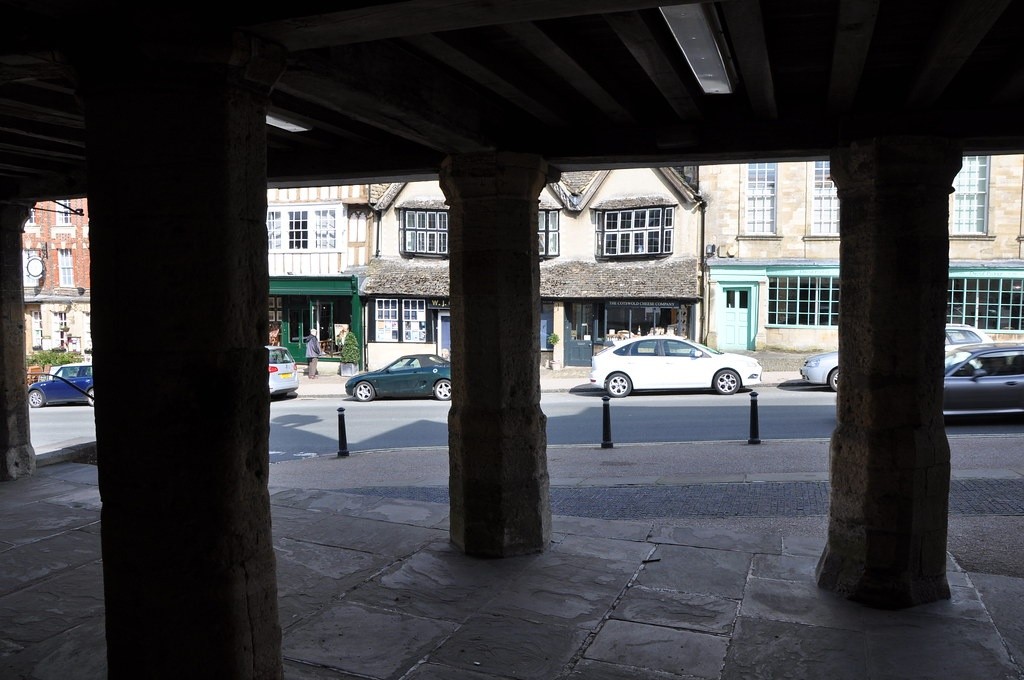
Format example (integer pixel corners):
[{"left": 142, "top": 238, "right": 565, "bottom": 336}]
[
  {"left": 27, "top": 362, "right": 95, "bottom": 408},
  {"left": 799, "top": 323, "right": 994, "bottom": 393},
  {"left": 589, "top": 335, "right": 763, "bottom": 398},
  {"left": 940, "top": 342, "right": 1024, "bottom": 416},
  {"left": 265, "top": 345, "right": 299, "bottom": 400},
  {"left": 344, "top": 354, "right": 452, "bottom": 402}
]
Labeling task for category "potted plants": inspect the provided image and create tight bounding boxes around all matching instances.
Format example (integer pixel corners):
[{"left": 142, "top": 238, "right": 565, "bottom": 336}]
[
  {"left": 340, "top": 331, "right": 361, "bottom": 376},
  {"left": 547, "top": 333, "right": 561, "bottom": 370}
]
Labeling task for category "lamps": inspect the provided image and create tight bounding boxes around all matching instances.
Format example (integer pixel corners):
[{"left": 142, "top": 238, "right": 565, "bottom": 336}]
[
  {"left": 707, "top": 244, "right": 715, "bottom": 256},
  {"left": 266, "top": 113, "right": 310, "bottom": 134},
  {"left": 658, "top": 0, "right": 737, "bottom": 94}
]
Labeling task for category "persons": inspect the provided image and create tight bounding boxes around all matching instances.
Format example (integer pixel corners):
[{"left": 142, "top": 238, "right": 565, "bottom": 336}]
[{"left": 306, "top": 329, "right": 322, "bottom": 379}]
